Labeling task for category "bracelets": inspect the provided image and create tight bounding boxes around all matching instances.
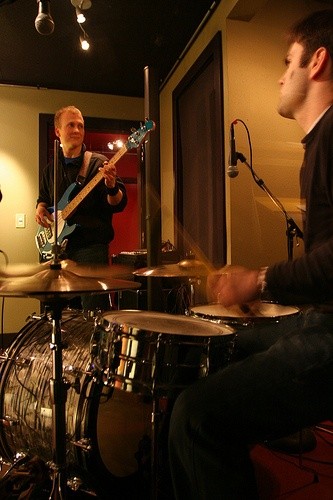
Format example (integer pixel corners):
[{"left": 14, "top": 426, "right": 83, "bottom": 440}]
[{"left": 256, "top": 266, "right": 267, "bottom": 295}]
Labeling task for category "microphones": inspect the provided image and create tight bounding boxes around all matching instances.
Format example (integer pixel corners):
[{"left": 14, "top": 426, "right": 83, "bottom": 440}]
[
  {"left": 226, "top": 123, "right": 239, "bottom": 177},
  {"left": 35, "top": 0, "right": 55, "bottom": 36}
]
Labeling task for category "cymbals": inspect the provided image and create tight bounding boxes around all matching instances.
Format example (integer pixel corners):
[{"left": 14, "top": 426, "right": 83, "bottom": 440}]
[
  {"left": 90, "top": 310, "right": 236, "bottom": 398},
  {"left": 191, "top": 301, "right": 299, "bottom": 367},
  {"left": 3, "top": 259, "right": 128, "bottom": 277},
  {"left": 133, "top": 257, "right": 214, "bottom": 278},
  {"left": 1, "top": 267, "right": 141, "bottom": 292}
]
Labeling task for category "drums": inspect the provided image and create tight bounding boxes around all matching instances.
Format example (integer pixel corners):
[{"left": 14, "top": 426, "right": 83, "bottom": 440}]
[{"left": 0, "top": 307, "right": 171, "bottom": 493}]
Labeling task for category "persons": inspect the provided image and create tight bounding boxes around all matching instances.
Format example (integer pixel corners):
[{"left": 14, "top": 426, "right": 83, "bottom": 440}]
[
  {"left": 166, "top": 10, "right": 333, "bottom": 500},
  {"left": 34, "top": 105, "right": 128, "bottom": 312}
]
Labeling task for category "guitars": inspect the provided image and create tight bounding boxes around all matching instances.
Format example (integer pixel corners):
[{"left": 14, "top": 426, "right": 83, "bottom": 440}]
[{"left": 34, "top": 117, "right": 155, "bottom": 260}]
